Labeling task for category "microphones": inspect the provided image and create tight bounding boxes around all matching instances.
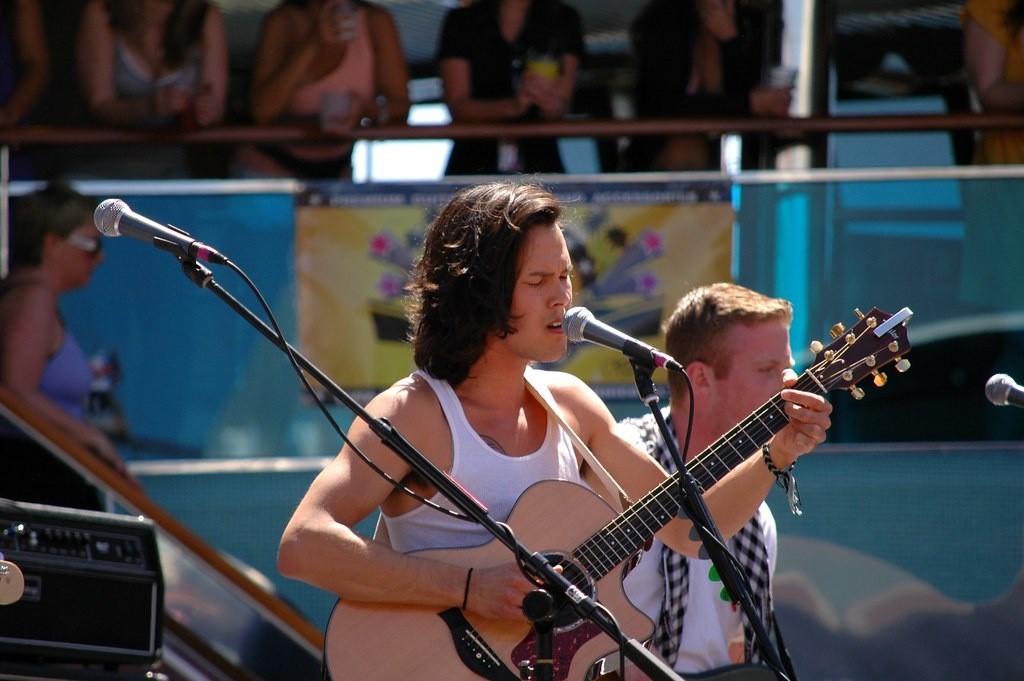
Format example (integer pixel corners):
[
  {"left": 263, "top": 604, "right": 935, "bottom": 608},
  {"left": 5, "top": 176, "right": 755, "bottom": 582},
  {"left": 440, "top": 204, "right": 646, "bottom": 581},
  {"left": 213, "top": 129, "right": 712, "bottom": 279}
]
[
  {"left": 94, "top": 199, "right": 229, "bottom": 266},
  {"left": 560, "top": 306, "right": 684, "bottom": 374},
  {"left": 984, "top": 373, "right": 1024, "bottom": 408}
]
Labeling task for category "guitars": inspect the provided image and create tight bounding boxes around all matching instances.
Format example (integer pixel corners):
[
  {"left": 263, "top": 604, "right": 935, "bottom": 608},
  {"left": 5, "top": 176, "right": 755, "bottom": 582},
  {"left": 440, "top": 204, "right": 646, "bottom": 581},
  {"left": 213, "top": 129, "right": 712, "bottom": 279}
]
[{"left": 324, "top": 304, "right": 915, "bottom": 681}]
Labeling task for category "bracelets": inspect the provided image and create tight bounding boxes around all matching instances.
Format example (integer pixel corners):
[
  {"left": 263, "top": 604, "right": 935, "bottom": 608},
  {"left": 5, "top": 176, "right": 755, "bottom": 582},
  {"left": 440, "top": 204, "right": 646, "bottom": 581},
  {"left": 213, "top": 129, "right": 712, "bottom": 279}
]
[
  {"left": 463, "top": 567, "right": 473, "bottom": 608},
  {"left": 762, "top": 442, "right": 803, "bottom": 516}
]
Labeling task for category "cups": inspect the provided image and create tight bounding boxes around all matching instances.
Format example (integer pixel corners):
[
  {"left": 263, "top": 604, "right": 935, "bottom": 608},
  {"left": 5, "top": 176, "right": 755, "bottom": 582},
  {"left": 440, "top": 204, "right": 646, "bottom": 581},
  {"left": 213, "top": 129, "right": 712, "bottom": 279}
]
[
  {"left": 759, "top": 63, "right": 798, "bottom": 89},
  {"left": 526, "top": 49, "right": 560, "bottom": 78},
  {"left": 332, "top": 0, "right": 357, "bottom": 40}
]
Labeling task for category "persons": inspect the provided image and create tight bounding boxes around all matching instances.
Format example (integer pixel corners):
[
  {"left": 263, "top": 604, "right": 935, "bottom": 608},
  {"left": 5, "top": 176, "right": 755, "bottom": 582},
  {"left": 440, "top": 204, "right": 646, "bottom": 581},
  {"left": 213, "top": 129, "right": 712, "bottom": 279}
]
[
  {"left": 228, "top": 0, "right": 410, "bottom": 179},
  {"left": 0, "top": 0, "right": 48, "bottom": 180},
  {"left": 278, "top": 183, "right": 833, "bottom": 622},
  {"left": 959, "top": 0, "right": 1024, "bottom": 165},
  {"left": 630, "top": 0, "right": 795, "bottom": 172},
  {"left": 438, "top": 1, "right": 586, "bottom": 174},
  {"left": 0, "top": 175, "right": 134, "bottom": 508},
  {"left": 620, "top": 281, "right": 795, "bottom": 681},
  {"left": 76, "top": 0, "right": 228, "bottom": 178}
]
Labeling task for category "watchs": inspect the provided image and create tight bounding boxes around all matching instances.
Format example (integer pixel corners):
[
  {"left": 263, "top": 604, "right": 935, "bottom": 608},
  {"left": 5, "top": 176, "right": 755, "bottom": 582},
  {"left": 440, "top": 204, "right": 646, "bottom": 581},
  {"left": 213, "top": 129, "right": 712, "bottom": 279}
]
[{"left": 357, "top": 95, "right": 389, "bottom": 143}]
